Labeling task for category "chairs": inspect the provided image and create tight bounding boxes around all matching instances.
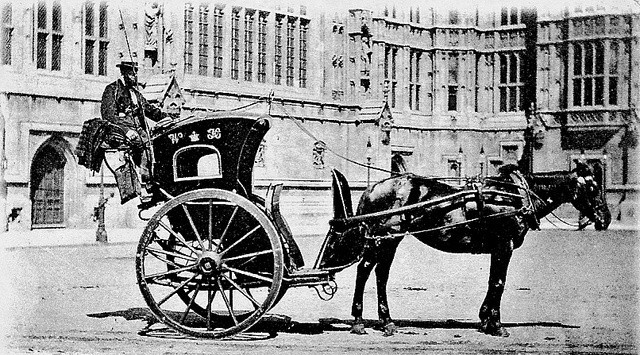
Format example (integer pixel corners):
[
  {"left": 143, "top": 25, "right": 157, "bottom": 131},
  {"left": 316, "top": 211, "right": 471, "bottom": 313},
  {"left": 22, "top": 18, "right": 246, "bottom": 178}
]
[{"left": 89, "top": 116, "right": 166, "bottom": 221}]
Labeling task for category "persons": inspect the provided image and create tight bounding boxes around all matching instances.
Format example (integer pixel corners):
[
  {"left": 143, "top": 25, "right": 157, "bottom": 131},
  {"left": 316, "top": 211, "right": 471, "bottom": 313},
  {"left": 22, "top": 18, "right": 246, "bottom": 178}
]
[{"left": 100, "top": 62, "right": 173, "bottom": 196}]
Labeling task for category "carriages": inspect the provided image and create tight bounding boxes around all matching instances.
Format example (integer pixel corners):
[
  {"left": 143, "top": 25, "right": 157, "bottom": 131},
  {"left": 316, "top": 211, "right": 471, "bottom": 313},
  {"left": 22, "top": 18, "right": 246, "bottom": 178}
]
[{"left": 97, "top": 91, "right": 613, "bottom": 338}]
